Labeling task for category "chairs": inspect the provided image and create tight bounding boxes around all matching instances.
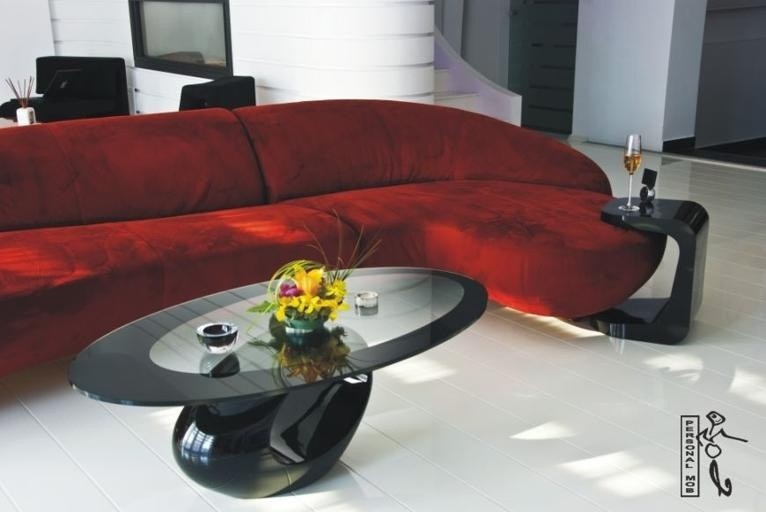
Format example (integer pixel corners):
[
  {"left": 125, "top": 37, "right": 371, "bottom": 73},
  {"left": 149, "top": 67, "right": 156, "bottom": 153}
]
[
  {"left": 1, "top": 56, "right": 127, "bottom": 128},
  {"left": 177, "top": 75, "right": 257, "bottom": 111}
]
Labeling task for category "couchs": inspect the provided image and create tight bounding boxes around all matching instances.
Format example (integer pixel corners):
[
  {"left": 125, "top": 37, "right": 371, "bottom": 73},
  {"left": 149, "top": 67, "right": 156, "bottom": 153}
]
[{"left": 0, "top": 101, "right": 671, "bottom": 374}]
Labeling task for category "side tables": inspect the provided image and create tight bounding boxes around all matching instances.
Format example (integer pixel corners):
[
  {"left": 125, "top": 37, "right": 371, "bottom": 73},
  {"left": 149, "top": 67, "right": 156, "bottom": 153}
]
[{"left": 593, "top": 202, "right": 707, "bottom": 343}]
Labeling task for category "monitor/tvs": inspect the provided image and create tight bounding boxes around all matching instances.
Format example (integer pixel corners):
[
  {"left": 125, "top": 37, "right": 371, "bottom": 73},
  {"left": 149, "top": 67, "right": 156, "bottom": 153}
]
[{"left": 133, "top": 0, "right": 231, "bottom": 75}]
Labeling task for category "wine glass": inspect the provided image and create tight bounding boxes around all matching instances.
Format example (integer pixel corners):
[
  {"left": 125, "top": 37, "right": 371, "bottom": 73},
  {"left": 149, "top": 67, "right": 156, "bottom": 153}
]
[{"left": 616, "top": 133, "right": 643, "bottom": 212}]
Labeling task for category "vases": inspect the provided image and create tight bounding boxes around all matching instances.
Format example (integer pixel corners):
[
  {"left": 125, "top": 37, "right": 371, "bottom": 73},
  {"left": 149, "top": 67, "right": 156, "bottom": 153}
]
[{"left": 278, "top": 311, "right": 330, "bottom": 329}]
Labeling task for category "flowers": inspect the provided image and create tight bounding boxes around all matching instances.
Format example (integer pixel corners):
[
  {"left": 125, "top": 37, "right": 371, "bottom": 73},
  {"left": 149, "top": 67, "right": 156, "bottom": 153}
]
[{"left": 248, "top": 209, "right": 385, "bottom": 324}]
